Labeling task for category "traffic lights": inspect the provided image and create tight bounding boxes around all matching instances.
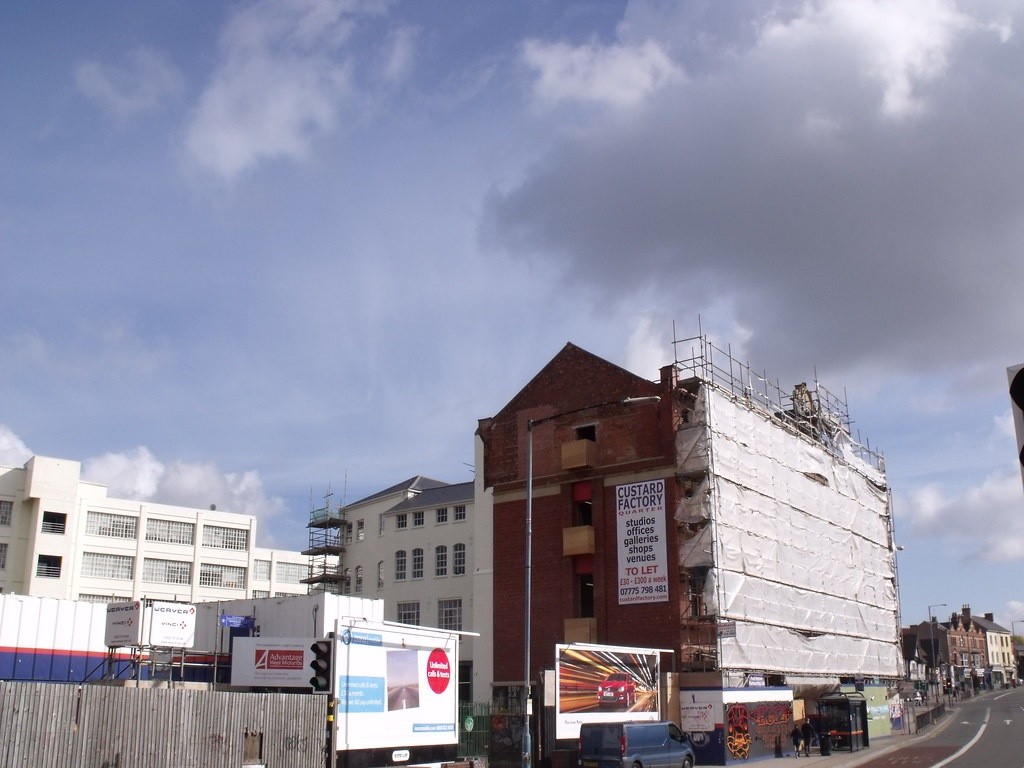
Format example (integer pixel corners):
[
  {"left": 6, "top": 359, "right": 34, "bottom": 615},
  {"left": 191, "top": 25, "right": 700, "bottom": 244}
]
[
  {"left": 308, "top": 637, "right": 335, "bottom": 696},
  {"left": 946, "top": 678, "right": 951, "bottom": 687}
]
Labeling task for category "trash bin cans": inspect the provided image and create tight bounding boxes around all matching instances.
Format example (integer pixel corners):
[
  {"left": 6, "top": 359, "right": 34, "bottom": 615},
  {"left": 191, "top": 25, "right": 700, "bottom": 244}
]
[{"left": 819, "top": 731, "right": 832, "bottom": 756}]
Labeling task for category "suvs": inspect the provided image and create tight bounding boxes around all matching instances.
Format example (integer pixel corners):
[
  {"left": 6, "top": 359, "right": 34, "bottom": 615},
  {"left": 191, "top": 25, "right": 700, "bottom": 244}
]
[{"left": 598, "top": 672, "right": 638, "bottom": 709}]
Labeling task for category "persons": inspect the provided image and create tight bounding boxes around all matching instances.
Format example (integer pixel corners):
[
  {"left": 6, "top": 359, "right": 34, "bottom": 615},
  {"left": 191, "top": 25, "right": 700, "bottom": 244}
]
[
  {"left": 801, "top": 718, "right": 815, "bottom": 757},
  {"left": 791, "top": 725, "right": 802, "bottom": 758}
]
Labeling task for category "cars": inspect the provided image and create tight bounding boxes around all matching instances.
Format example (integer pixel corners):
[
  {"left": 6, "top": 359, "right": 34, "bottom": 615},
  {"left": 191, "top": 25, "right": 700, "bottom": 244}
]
[{"left": 1018, "top": 678, "right": 1023, "bottom": 684}]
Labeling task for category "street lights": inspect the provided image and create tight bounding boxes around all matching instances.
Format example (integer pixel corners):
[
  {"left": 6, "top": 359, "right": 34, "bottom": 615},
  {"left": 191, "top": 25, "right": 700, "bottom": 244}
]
[
  {"left": 1010, "top": 618, "right": 1024, "bottom": 663},
  {"left": 927, "top": 603, "right": 948, "bottom": 718}
]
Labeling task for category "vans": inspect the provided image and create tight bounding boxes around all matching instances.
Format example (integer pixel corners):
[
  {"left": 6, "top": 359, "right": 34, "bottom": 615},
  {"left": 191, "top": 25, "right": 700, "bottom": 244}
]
[{"left": 577, "top": 719, "right": 698, "bottom": 768}]
